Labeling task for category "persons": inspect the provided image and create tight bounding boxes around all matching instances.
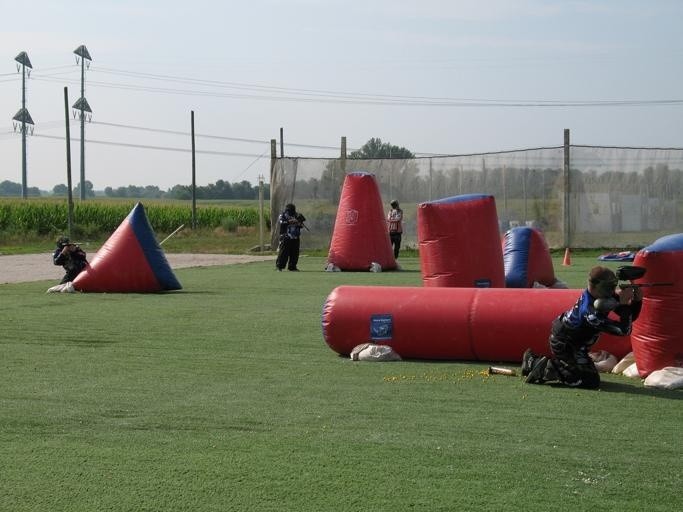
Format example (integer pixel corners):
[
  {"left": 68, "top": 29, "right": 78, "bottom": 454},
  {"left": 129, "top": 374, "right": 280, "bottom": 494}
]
[
  {"left": 516, "top": 263, "right": 645, "bottom": 391},
  {"left": 273, "top": 203, "right": 303, "bottom": 273},
  {"left": 50, "top": 236, "right": 89, "bottom": 286},
  {"left": 384, "top": 199, "right": 403, "bottom": 260}
]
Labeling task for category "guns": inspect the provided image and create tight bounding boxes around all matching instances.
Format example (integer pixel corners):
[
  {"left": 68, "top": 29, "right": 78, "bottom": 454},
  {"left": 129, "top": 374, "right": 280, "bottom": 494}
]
[
  {"left": 60, "top": 242, "right": 87, "bottom": 249},
  {"left": 294, "top": 212, "right": 311, "bottom": 234},
  {"left": 618, "top": 282, "right": 674, "bottom": 303}
]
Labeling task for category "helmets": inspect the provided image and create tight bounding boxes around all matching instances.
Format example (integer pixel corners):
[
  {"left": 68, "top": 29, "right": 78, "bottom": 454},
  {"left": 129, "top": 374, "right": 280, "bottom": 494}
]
[
  {"left": 588, "top": 267, "right": 618, "bottom": 297},
  {"left": 390, "top": 199, "right": 400, "bottom": 209},
  {"left": 56, "top": 238, "right": 69, "bottom": 248},
  {"left": 285, "top": 203, "right": 296, "bottom": 213}
]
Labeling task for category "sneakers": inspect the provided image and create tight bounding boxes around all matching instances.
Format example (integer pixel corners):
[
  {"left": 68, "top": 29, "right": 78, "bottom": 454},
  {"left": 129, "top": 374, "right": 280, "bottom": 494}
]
[{"left": 521, "top": 347, "right": 549, "bottom": 383}]
